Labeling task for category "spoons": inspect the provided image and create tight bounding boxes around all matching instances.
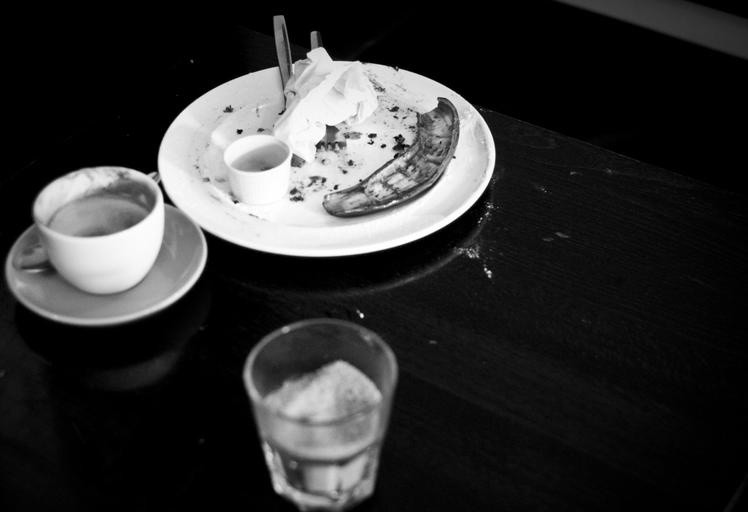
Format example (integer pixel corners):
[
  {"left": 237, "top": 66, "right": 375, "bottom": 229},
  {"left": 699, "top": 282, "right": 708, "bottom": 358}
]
[{"left": 13, "top": 171, "right": 164, "bottom": 273}]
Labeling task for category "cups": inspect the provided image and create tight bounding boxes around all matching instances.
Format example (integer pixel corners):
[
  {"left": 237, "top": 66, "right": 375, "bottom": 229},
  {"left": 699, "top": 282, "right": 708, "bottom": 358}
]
[
  {"left": 241, "top": 318, "right": 400, "bottom": 511},
  {"left": 32, "top": 164, "right": 164, "bottom": 296}
]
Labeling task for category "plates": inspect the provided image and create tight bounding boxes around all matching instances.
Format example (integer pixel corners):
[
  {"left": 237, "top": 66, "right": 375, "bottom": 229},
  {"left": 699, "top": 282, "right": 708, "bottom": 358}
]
[
  {"left": 3, "top": 202, "right": 210, "bottom": 329},
  {"left": 156, "top": 59, "right": 497, "bottom": 260}
]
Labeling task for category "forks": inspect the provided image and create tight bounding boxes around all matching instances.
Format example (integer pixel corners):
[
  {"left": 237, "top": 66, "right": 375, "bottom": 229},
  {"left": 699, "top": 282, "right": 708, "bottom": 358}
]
[{"left": 309, "top": 30, "right": 346, "bottom": 151}]
